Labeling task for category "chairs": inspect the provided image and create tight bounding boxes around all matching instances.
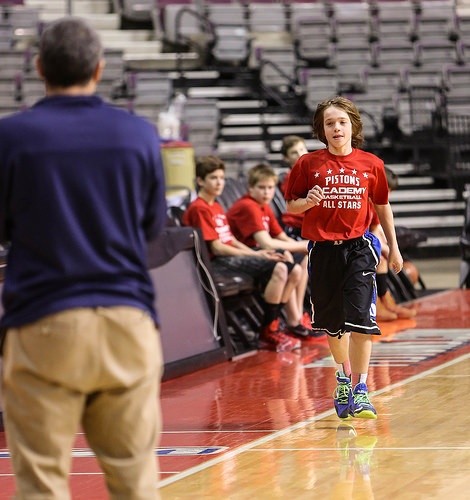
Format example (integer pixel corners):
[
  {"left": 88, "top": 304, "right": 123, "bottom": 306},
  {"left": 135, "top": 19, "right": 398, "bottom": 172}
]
[{"left": 0, "top": 0, "right": 470, "bottom": 362}]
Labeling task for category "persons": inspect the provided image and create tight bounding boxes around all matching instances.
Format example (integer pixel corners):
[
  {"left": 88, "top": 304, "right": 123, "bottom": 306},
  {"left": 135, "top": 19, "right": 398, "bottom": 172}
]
[
  {"left": 182, "top": 97, "right": 404, "bottom": 421},
  {"left": 0, "top": 16, "right": 168, "bottom": 500}
]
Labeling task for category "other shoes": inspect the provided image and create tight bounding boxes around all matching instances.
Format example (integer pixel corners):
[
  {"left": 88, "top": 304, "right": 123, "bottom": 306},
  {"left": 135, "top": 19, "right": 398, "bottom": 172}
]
[
  {"left": 280, "top": 320, "right": 329, "bottom": 341},
  {"left": 257, "top": 327, "right": 302, "bottom": 352},
  {"left": 376, "top": 293, "right": 417, "bottom": 320}
]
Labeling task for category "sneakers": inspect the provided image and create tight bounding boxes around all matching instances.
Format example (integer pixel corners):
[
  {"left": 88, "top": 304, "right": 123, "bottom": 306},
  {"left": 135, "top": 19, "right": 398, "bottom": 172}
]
[
  {"left": 332, "top": 370, "right": 351, "bottom": 417},
  {"left": 349, "top": 382, "right": 377, "bottom": 419}
]
